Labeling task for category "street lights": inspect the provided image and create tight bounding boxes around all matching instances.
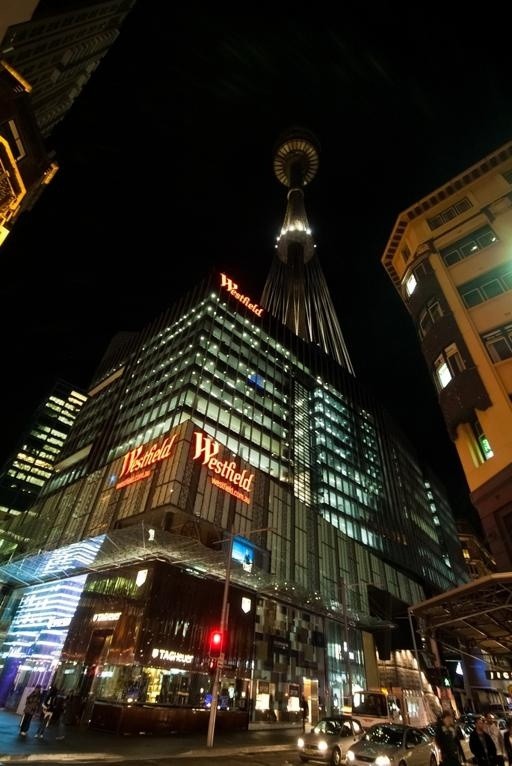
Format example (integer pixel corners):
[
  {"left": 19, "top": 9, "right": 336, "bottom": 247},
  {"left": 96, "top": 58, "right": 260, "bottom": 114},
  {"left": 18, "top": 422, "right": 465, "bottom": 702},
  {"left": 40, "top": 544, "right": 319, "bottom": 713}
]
[
  {"left": 205, "top": 526, "right": 278, "bottom": 748},
  {"left": 340, "top": 579, "right": 382, "bottom": 709}
]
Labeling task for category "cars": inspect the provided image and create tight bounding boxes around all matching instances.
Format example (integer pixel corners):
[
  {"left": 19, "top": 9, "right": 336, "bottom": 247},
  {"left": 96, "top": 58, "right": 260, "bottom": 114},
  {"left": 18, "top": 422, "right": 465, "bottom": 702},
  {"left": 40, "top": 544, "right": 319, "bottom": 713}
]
[
  {"left": 346, "top": 722, "right": 441, "bottom": 766},
  {"left": 298, "top": 717, "right": 365, "bottom": 764}
]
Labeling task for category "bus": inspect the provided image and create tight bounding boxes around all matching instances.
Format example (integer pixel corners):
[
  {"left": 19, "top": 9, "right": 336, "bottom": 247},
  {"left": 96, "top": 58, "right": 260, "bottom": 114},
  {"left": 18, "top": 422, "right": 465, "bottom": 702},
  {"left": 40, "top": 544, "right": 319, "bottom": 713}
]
[{"left": 349, "top": 689, "right": 435, "bottom": 731}]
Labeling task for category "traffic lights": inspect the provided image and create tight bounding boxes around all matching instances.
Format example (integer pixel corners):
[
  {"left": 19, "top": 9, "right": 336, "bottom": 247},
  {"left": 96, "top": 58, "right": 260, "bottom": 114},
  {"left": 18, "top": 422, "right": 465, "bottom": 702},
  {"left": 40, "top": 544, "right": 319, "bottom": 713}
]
[{"left": 210, "top": 632, "right": 223, "bottom": 659}]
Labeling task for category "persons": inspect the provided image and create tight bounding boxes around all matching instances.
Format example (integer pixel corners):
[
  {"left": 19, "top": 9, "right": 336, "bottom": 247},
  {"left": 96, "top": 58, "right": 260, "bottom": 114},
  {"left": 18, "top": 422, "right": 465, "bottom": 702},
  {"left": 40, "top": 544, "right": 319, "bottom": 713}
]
[
  {"left": 17, "top": 685, "right": 43, "bottom": 736},
  {"left": 482, "top": 713, "right": 509, "bottom": 766},
  {"left": 468, "top": 717, "right": 498, "bottom": 765},
  {"left": 435, "top": 710, "right": 463, "bottom": 766},
  {"left": 503, "top": 718, "right": 512, "bottom": 766},
  {"left": 34, "top": 688, "right": 59, "bottom": 739}
]
